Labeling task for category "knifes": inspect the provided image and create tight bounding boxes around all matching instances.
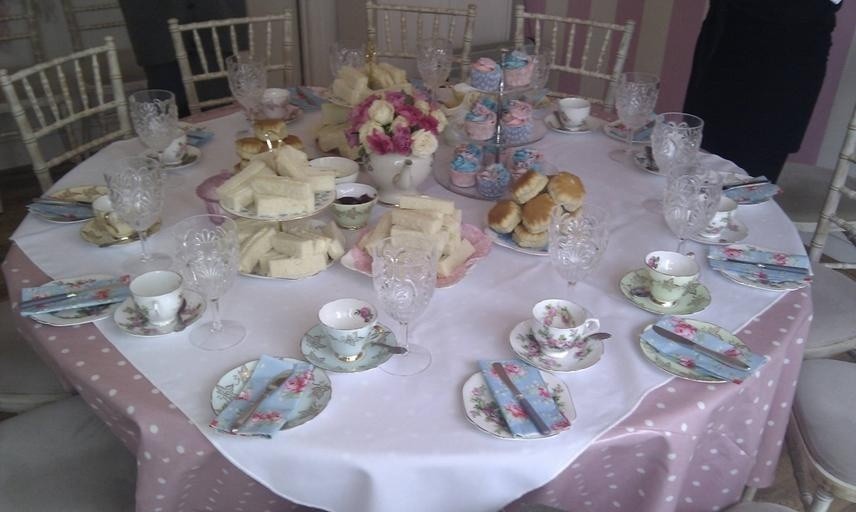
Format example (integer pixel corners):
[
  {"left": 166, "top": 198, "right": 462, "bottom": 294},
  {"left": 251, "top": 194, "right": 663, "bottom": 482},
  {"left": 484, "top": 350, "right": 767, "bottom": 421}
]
[
  {"left": 650, "top": 324, "right": 752, "bottom": 372},
  {"left": 232, "top": 361, "right": 298, "bottom": 432},
  {"left": 723, "top": 179, "right": 770, "bottom": 191},
  {"left": 728, "top": 256, "right": 809, "bottom": 275},
  {"left": 14, "top": 285, "right": 122, "bottom": 310},
  {"left": 492, "top": 362, "right": 550, "bottom": 434},
  {"left": 31, "top": 197, "right": 90, "bottom": 208}
]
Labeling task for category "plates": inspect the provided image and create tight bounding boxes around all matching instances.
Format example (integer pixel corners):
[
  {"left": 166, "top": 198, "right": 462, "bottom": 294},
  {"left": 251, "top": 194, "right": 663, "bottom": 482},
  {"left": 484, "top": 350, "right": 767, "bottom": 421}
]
[
  {"left": 483, "top": 223, "right": 549, "bottom": 260},
  {"left": 299, "top": 322, "right": 397, "bottom": 374},
  {"left": 638, "top": 316, "right": 755, "bottom": 384},
  {"left": 509, "top": 319, "right": 607, "bottom": 374},
  {"left": 432, "top": 142, "right": 560, "bottom": 201},
  {"left": 136, "top": 144, "right": 204, "bottom": 171},
  {"left": 218, "top": 189, "right": 335, "bottom": 223},
  {"left": 208, "top": 356, "right": 333, "bottom": 438},
  {"left": 461, "top": 368, "right": 577, "bottom": 441},
  {"left": 339, "top": 218, "right": 493, "bottom": 290},
  {"left": 690, "top": 220, "right": 751, "bottom": 246},
  {"left": 79, "top": 212, "right": 160, "bottom": 248},
  {"left": 725, "top": 171, "right": 772, "bottom": 206},
  {"left": 617, "top": 268, "right": 713, "bottom": 317},
  {"left": 543, "top": 113, "right": 594, "bottom": 135},
  {"left": 447, "top": 110, "right": 546, "bottom": 145},
  {"left": 630, "top": 150, "right": 748, "bottom": 179},
  {"left": 712, "top": 244, "right": 813, "bottom": 292},
  {"left": 288, "top": 85, "right": 328, "bottom": 110},
  {"left": 237, "top": 218, "right": 348, "bottom": 283},
  {"left": 29, "top": 186, "right": 109, "bottom": 224},
  {"left": 23, "top": 275, "right": 139, "bottom": 329},
  {"left": 605, "top": 120, "right": 654, "bottom": 147}
]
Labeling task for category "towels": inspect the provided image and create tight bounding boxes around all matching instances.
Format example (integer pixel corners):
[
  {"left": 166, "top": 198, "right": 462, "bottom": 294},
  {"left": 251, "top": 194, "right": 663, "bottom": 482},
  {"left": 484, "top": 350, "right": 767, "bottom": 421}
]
[
  {"left": 706, "top": 236, "right": 811, "bottom": 290},
  {"left": 638, "top": 315, "right": 773, "bottom": 387},
  {"left": 280, "top": 87, "right": 322, "bottom": 111},
  {"left": 476, "top": 355, "right": 567, "bottom": 447},
  {"left": 177, "top": 126, "right": 216, "bottom": 151},
  {"left": 709, "top": 171, "right": 783, "bottom": 211},
  {"left": 205, "top": 353, "right": 315, "bottom": 442},
  {"left": 8, "top": 273, "right": 133, "bottom": 320},
  {"left": 28, "top": 192, "right": 97, "bottom": 225}
]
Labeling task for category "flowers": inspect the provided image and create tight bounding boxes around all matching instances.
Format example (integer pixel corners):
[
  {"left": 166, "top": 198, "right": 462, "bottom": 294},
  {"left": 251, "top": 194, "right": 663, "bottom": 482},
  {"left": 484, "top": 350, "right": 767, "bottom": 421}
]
[{"left": 321, "top": 57, "right": 449, "bottom": 165}]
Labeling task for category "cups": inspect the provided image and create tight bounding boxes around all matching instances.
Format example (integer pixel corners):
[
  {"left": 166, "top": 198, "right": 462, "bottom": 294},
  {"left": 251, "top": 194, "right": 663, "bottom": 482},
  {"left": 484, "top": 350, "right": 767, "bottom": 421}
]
[
  {"left": 700, "top": 196, "right": 739, "bottom": 237},
  {"left": 527, "top": 298, "right": 601, "bottom": 359},
  {"left": 91, "top": 195, "right": 139, "bottom": 236},
  {"left": 524, "top": 46, "right": 555, "bottom": 90},
  {"left": 319, "top": 298, "right": 377, "bottom": 364}
]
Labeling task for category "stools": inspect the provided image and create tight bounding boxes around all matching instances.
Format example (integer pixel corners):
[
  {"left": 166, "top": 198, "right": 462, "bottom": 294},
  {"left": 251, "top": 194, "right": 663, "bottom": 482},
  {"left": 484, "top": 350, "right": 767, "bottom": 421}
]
[
  {"left": 0, "top": 391, "right": 139, "bottom": 512},
  {"left": 0, "top": 297, "right": 78, "bottom": 414},
  {"left": 740, "top": 355, "right": 855, "bottom": 512}
]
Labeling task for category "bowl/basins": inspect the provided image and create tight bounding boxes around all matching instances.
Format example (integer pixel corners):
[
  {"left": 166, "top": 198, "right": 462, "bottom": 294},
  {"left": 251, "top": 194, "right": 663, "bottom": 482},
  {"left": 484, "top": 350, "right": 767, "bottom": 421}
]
[
  {"left": 309, "top": 154, "right": 362, "bottom": 189},
  {"left": 644, "top": 250, "right": 701, "bottom": 305},
  {"left": 328, "top": 183, "right": 380, "bottom": 231},
  {"left": 260, "top": 86, "right": 290, "bottom": 120},
  {"left": 556, "top": 98, "right": 593, "bottom": 126}
]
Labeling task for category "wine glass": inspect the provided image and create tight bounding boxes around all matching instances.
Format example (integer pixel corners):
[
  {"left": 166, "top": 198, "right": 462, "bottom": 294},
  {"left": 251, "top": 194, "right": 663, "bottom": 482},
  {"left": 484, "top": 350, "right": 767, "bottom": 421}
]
[
  {"left": 370, "top": 223, "right": 439, "bottom": 377},
  {"left": 103, "top": 156, "right": 166, "bottom": 263},
  {"left": 417, "top": 36, "right": 454, "bottom": 102},
  {"left": 609, "top": 72, "right": 660, "bottom": 163},
  {"left": 652, "top": 110, "right": 704, "bottom": 212},
  {"left": 226, "top": 51, "right": 263, "bottom": 128},
  {"left": 660, "top": 166, "right": 720, "bottom": 253},
  {"left": 171, "top": 212, "right": 249, "bottom": 350},
  {"left": 128, "top": 88, "right": 178, "bottom": 156},
  {"left": 546, "top": 202, "right": 612, "bottom": 297}
]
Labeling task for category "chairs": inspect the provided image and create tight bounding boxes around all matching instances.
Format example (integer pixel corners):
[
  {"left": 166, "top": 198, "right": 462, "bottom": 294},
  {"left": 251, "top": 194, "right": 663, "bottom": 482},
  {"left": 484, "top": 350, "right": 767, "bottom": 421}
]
[
  {"left": 357, "top": 0, "right": 481, "bottom": 91},
  {"left": 163, "top": 9, "right": 297, "bottom": 121},
  {"left": 788, "top": 100, "right": 855, "bottom": 361},
  {"left": 0, "top": 33, "right": 133, "bottom": 199},
  {"left": 505, "top": 0, "right": 639, "bottom": 117}
]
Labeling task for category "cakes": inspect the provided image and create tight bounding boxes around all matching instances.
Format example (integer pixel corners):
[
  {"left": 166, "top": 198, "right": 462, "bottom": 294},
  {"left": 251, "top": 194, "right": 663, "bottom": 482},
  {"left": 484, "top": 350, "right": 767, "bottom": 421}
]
[{"left": 452, "top": 51, "right": 544, "bottom": 198}]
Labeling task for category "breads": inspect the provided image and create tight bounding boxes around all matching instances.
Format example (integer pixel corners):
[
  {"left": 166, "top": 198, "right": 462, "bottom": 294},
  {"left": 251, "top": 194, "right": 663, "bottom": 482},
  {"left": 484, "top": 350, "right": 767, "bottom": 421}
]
[
  {"left": 359, "top": 194, "right": 475, "bottom": 278},
  {"left": 217, "top": 143, "right": 337, "bottom": 220},
  {"left": 316, "top": 101, "right": 363, "bottom": 160},
  {"left": 487, "top": 170, "right": 588, "bottom": 251},
  {"left": 220, "top": 216, "right": 345, "bottom": 278},
  {"left": 330, "top": 63, "right": 409, "bottom": 103},
  {"left": 233, "top": 118, "right": 306, "bottom": 171}
]
